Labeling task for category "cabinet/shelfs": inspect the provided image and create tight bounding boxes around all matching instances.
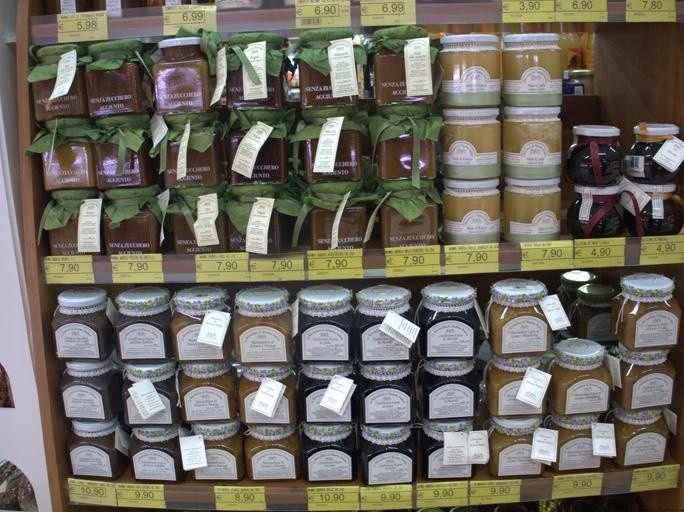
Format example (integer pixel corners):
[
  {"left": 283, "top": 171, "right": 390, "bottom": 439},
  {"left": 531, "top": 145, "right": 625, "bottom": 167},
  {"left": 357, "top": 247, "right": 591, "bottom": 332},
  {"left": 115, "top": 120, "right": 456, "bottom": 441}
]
[{"left": 14, "top": 0, "right": 684, "bottom": 511}]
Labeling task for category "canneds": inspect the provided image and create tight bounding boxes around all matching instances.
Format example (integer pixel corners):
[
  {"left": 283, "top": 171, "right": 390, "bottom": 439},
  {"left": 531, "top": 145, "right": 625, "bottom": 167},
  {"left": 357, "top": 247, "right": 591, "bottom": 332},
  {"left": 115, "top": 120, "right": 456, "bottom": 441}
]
[
  {"left": 567, "top": 68, "right": 596, "bottom": 95},
  {"left": 300, "top": 284, "right": 356, "bottom": 368},
  {"left": 362, "top": 422, "right": 418, "bottom": 484},
  {"left": 369, "top": 27, "right": 432, "bottom": 108},
  {"left": 504, "top": 30, "right": 565, "bottom": 107},
  {"left": 487, "top": 417, "right": 547, "bottom": 477},
  {"left": 570, "top": 125, "right": 627, "bottom": 185},
  {"left": 170, "top": 286, "right": 232, "bottom": 363},
  {"left": 632, "top": 125, "right": 680, "bottom": 186},
  {"left": 299, "top": 359, "right": 358, "bottom": 426},
  {"left": 420, "top": 421, "right": 473, "bottom": 479},
  {"left": 165, "top": 187, "right": 223, "bottom": 254},
  {"left": 554, "top": 338, "right": 614, "bottom": 417},
  {"left": 37, "top": 117, "right": 93, "bottom": 185},
  {"left": 539, "top": 414, "right": 604, "bottom": 471},
  {"left": 53, "top": 285, "right": 111, "bottom": 359},
  {"left": 606, "top": 407, "right": 672, "bottom": 467},
  {"left": 177, "top": 362, "right": 237, "bottom": 427},
  {"left": 242, "top": 423, "right": 299, "bottom": 479},
  {"left": 352, "top": 286, "right": 416, "bottom": 366},
  {"left": 628, "top": 184, "right": 681, "bottom": 234},
  {"left": 151, "top": 35, "right": 216, "bottom": 112},
  {"left": 560, "top": 272, "right": 593, "bottom": 314},
  {"left": 116, "top": 289, "right": 168, "bottom": 364},
  {"left": 186, "top": 424, "right": 244, "bottom": 482},
  {"left": 445, "top": 180, "right": 502, "bottom": 243},
  {"left": 570, "top": 188, "right": 621, "bottom": 236},
  {"left": 506, "top": 179, "right": 561, "bottom": 241},
  {"left": 65, "top": 421, "right": 120, "bottom": 477},
  {"left": 424, "top": 358, "right": 482, "bottom": 421},
  {"left": 130, "top": 426, "right": 185, "bottom": 484},
  {"left": 503, "top": 104, "right": 568, "bottom": 180},
  {"left": 106, "top": 186, "right": 161, "bottom": 254},
  {"left": 157, "top": 112, "right": 219, "bottom": 187},
  {"left": 613, "top": 344, "right": 673, "bottom": 406},
  {"left": 613, "top": 274, "right": 678, "bottom": 352},
  {"left": 302, "top": 422, "right": 356, "bottom": 480},
  {"left": 419, "top": 282, "right": 481, "bottom": 360},
  {"left": 296, "top": 28, "right": 364, "bottom": 106},
  {"left": 30, "top": 44, "right": 89, "bottom": 118},
  {"left": 302, "top": 110, "right": 363, "bottom": 185},
  {"left": 44, "top": 190, "right": 95, "bottom": 254},
  {"left": 361, "top": 363, "right": 417, "bottom": 427},
  {"left": 84, "top": 39, "right": 144, "bottom": 116},
  {"left": 217, "top": 187, "right": 286, "bottom": 251},
  {"left": 441, "top": 34, "right": 502, "bottom": 109},
  {"left": 58, "top": 361, "right": 121, "bottom": 424},
  {"left": 226, "top": 31, "right": 289, "bottom": 109},
  {"left": 235, "top": 284, "right": 293, "bottom": 368},
  {"left": 92, "top": 111, "right": 150, "bottom": 188},
  {"left": 572, "top": 284, "right": 616, "bottom": 347},
  {"left": 221, "top": 107, "right": 291, "bottom": 186},
  {"left": 377, "top": 180, "right": 438, "bottom": 246},
  {"left": 117, "top": 362, "right": 182, "bottom": 427},
  {"left": 490, "top": 278, "right": 551, "bottom": 360},
  {"left": 371, "top": 108, "right": 439, "bottom": 186},
  {"left": 485, "top": 358, "right": 542, "bottom": 417},
  {"left": 444, "top": 108, "right": 506, "bottom": 177},
  {"left": 302, "top": 184, "right": 368, "bottom": 248},
  {"left": 234, "top": 366, "right": 299, "bottom": 426}
]
